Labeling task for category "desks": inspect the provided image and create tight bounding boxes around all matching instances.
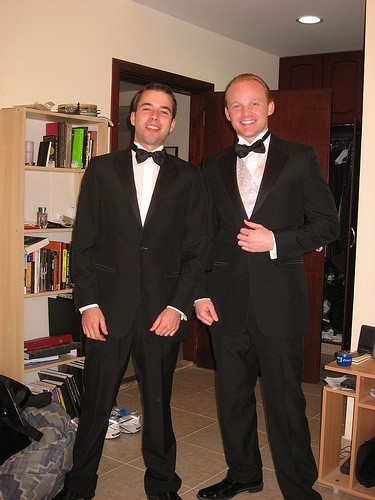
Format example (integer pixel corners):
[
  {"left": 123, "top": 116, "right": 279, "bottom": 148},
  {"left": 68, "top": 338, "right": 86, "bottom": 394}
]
[{"left": 317, "top": 351, "right": 375, "bottom": 500}]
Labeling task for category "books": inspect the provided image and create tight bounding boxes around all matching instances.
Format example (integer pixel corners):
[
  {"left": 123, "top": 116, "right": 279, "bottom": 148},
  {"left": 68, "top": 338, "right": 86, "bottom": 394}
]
[
  {"left": 24, "top": 236, "right": 74, "bottom": 293},
  {"left": 24, "top": 334, "right": 82, "bottom": 366},
  {"left": 28, "top": 359, "right": 85, "bottom": 420},
  {"left": 38, "top": 121, "right": 97, "bottom": 169}
]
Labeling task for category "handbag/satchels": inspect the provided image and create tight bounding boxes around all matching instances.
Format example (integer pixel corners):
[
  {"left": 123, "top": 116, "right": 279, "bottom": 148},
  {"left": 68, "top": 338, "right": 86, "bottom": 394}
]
[{"left": 355, "top": 437, "right": 375, "bottom": 487}]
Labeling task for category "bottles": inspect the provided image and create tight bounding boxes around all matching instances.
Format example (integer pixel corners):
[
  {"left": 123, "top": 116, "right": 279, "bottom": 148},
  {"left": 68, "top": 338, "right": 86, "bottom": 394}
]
[{"left": 39, "top": 207, "right": 48, "bottom": 229}]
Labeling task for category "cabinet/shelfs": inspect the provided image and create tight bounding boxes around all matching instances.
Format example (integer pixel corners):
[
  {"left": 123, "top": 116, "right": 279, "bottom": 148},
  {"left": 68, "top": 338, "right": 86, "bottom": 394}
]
[
  {"left": 278, "top": 50, "right": 364, "bottom": 124},
  {"left": 321, "top": 117, "right": 361, "bottom": 350},
  {"left": 0, "top": 107, "right": 108, "bottom": 384}
]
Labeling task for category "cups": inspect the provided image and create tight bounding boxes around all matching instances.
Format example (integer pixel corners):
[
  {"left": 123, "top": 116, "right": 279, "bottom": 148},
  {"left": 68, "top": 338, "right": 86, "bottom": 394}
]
[{"left": 25, "top": 141, "right": 34, "bottom": 167}]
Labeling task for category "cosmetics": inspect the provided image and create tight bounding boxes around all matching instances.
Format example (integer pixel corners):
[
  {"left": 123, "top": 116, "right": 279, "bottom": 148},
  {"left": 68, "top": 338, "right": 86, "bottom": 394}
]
[
  {"left": 37, "top": 207, "right": 48, "bottom": 228},
  {"left": 25, "top": 141, "right": 34, "bottom": 165}
]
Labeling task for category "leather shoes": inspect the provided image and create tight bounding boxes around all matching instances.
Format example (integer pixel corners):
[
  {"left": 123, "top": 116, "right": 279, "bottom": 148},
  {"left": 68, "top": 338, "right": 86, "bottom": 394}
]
[{"left": 196, "top": 473, "right": 264, "bottom": 500}]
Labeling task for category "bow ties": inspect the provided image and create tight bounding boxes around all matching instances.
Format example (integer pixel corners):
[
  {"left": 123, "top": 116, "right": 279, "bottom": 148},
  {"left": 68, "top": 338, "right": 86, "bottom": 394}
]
[
  {"left": 130, "top": 141, "right": 166, "bottom": 167},
  {"left": 234, "top": 129, "right": 271, "bottom": 158}
]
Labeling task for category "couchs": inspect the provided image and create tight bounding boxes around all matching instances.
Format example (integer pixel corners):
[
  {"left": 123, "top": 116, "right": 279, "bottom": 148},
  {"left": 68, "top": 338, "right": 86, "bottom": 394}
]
[{"left": 0, "top": 392, "right": 77, "bottom": 500}]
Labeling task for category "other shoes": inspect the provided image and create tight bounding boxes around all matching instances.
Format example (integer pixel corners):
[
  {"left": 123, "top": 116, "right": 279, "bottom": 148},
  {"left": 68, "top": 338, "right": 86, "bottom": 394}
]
[
  {"left": 147, "top": 490, "right": 182, "bottom": 500},
  {"left": 53, "top": 487, "right": 91, "bottom": 500}
]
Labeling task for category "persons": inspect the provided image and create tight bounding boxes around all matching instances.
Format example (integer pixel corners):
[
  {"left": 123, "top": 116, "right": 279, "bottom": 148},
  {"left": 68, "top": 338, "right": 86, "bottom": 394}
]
[
  {"left": 53, "top": 84, "right": 208, "bottom": 500},
  {"left": 192, "top": 73, "right": 340, "bottom": 500}
]
